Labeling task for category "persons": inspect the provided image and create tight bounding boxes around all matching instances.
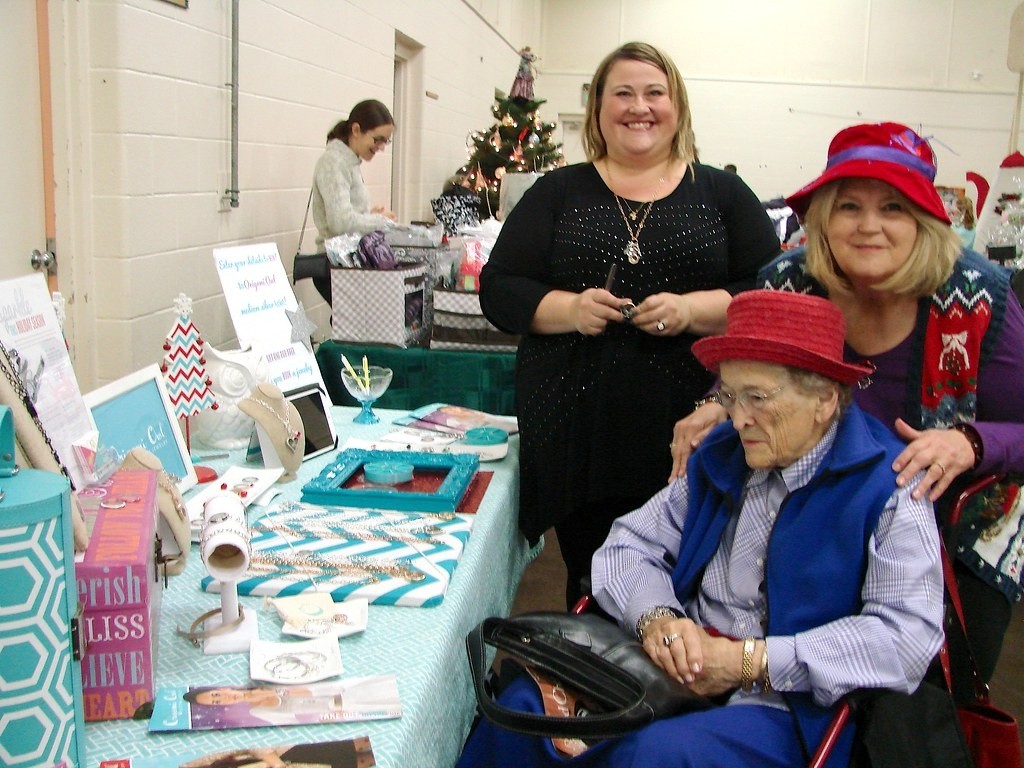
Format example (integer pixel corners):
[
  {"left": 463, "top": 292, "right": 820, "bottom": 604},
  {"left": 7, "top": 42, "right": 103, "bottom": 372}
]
[
  {"left": 183, "top": 674, "right": 401, "bottom": 725},
  {"left": 477, "top": 42, "right": 782, "bottom": 614},
  {"left": 312, "top": 100, "right": 397, "bottom": 326},
  {"left": 236, "top": 384, "right": 306, "bottom": 481},
  {"left": 950, "top": 198, "right": 976, "bottom": 249},
  {"left": 667, "top": 120, "right": 1024, "bottom": 709},
  {"left": 454, "top": 292, "right": 945, "bottom": 768}
]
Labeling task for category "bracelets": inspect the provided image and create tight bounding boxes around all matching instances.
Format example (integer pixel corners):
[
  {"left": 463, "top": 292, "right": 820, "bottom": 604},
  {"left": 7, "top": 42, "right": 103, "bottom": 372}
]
[
  {"left": 263, "top": 613, "right": 348, "bottom": 679},
  {"left": 636, "top": 607, "right": 678, "bottom": 644},
  {"left": 695, "top": 391, "right": 722, "bottom": 409},
  {"left": 197, "top": 493, "right": 253, "bottom": 570},
  {"left": 949, "top": 423, "right": 981, "bottom": 470},
  {"left": 761, "top": 638, "right": 770, "bottom": 694}
]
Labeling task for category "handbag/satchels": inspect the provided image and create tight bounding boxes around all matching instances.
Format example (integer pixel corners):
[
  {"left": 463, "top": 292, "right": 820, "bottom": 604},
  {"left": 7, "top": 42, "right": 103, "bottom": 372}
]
[
  {"left": 465, "top": 609, "right": 704, "bottom": 738},
  {"left": 293, "top": 253, "right": 331, "bottom": 292},
  {"left": 939, "top": 533, "right": 1024, "bottom": 768}
]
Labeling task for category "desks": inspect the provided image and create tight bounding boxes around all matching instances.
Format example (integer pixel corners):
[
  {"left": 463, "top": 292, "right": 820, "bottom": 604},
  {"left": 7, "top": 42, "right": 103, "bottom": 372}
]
[
  {"left": 313, "top": 338, "right": 520, "bottom": 417},
  {"left": 84, "top": 407, "right": 546, "bottom": 768}
]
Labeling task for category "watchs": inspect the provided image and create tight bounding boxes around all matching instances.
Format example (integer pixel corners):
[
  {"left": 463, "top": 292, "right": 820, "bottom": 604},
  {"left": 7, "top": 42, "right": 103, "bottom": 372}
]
[{"left": 742, "top": 636, "right": 755, "bottom": 693}]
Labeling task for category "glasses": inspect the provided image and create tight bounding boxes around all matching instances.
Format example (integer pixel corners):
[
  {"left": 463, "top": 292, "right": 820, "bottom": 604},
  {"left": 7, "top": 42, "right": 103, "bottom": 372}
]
[
  {"left": 712, "top": 368, "right": 801, "bottom": 412},
  {"left": 357, "top": 121, "right": 392, "bottom": 145}
]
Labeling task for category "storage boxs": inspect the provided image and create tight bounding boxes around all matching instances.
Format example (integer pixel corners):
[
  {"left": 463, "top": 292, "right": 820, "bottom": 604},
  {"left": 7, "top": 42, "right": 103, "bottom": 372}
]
[
  {"left": 72, "top": 468, "right": 162, "bottom": 725},
  {"left": 326, "top": 262, "right": 427, "bottom": 347}
]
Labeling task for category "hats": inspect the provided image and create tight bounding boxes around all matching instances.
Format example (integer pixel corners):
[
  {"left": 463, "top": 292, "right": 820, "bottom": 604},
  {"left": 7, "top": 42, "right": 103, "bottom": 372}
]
[
  {"left": 784, "top": 121, "right": 952, "bottom": 225},
  {"left": 691, "top": 289, "right": 873, "bottom": 386}
]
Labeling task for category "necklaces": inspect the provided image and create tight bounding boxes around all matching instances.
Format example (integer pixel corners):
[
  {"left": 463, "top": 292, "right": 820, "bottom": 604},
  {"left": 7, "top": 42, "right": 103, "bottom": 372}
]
[
  {"left": 247, "top": 394, "right": 301, "bottom": 452},
  {"left": 248, "top": 502, "right": 455, "bottom": 586},
  {"left": 604, "top": 153, "right": 673, "bottom": 265},
  {"left": 619, "top": 193, "right": 649, "bottom": 220}
]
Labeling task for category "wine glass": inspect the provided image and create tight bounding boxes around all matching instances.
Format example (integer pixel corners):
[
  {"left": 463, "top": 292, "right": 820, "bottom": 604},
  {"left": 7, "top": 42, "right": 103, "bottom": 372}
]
[{"left": 341, "top": 366, "right": 393, "bottom": 424}]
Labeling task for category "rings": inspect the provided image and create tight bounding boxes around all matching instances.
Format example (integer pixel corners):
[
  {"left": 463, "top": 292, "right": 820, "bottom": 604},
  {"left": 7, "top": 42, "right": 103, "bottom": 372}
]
[
  {"left": 663, "top": 633, "right": 682, "bottom": 646},
  {"left": 619, "top": 303, "right": 637, "bottom": 320},
  {"left": 656, "top": 320, "right": 665, "bottom": 331},
  {"left": 933, "top": 461, "right": 946, "bottom": 476},
  {"left": 668, "top": 443, "right": 678, "bottom": 448}
]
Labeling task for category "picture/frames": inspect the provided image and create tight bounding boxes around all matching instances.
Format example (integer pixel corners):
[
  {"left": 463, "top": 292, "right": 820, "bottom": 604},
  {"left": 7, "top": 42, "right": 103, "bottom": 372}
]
[{"left": 82, "top": 361, "right": 201, "bottom": 495}]
